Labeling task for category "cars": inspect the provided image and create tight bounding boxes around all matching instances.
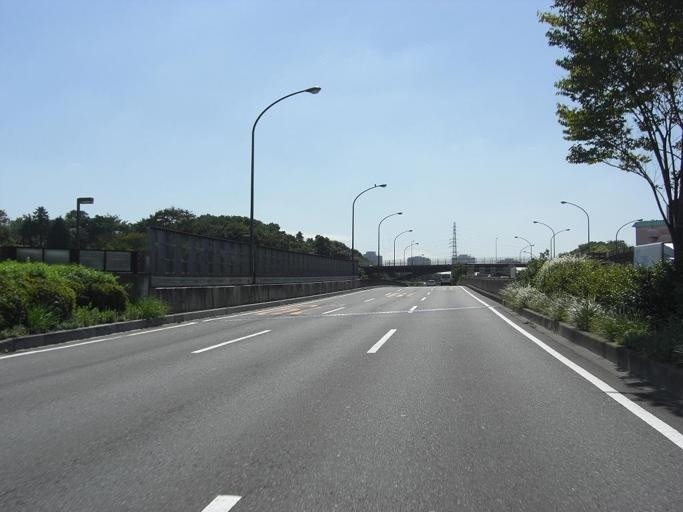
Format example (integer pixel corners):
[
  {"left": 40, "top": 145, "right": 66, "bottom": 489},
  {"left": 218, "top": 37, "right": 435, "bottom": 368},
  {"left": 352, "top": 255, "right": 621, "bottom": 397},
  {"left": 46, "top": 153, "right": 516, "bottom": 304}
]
[{"left": 426, "top": 279, "right": 436, "bottom": 286}]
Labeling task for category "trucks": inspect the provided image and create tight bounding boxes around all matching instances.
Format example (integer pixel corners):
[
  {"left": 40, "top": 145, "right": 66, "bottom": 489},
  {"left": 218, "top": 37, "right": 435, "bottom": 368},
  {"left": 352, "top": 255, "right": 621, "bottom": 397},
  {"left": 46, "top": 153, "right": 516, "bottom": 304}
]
[{"left": 630, "top": 243, "right": 675, "bottom": 267}]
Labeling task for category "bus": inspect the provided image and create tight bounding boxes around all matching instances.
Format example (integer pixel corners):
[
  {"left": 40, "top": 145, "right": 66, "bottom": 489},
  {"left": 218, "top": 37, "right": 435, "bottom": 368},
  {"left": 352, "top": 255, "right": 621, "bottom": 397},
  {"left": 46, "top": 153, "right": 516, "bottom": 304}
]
[{"left": 440, "top": 273, "right": 451, "bottom": 285}]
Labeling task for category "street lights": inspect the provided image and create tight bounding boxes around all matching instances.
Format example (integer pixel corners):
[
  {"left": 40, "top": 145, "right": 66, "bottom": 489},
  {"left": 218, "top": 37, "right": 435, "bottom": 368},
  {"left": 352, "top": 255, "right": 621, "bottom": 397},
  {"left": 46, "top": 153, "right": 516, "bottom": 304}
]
[
  {"left": 73, "top": 196, "right": 95, "bottom": 249},
  {"left": 519, "top": 251, "right": 536, "bottom": 260},
  {"left": 393, "top": 229, "right": 412, "bottom": 271},
  {"left": 350, "top": 183, "right": 386, "bottom": 279},
  {"left": 550, "top": 228, "right": 570, "bottom": 260},
  {"left": 403, "top": 242, "right": 418, "bottom": 271},
  {"left": 249, "top": 86, "right": 322, "bottom": 286},
  {"left": 377, "top": 211, "right": 402, "bottom": 277},
  {"left": 560, "top": 201, "right": 589, "bottom": 257},
  {"left": 517, "top": 243, "right": 533, "bottom": 262},
  {"left": 513, "top": 235, "right": 537, "bottom": 263},
  {"left": 615, "top": 218, "right": 643, "bottom": 264},
  {"left": 532, "top": 220, "right": 555, "bottom": 259}
]
